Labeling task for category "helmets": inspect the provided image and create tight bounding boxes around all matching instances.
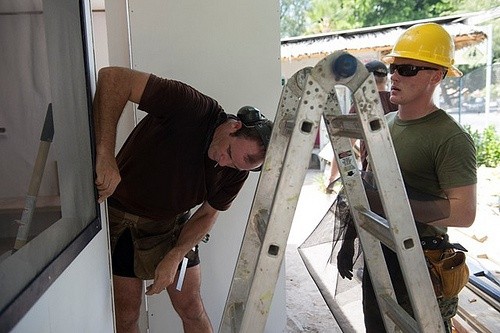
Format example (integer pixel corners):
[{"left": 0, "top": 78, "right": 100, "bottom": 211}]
[{"left": 387, "top": 23, "right": 463, "bottom": 77}]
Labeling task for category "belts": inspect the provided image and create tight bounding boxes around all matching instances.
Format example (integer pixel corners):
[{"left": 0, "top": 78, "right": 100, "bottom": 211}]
[{"left": 422, "top": 235, "right": 449, "bottom": 250}]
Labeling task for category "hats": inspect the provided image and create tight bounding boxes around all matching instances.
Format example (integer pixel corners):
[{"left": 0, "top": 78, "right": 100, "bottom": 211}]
[{"left": 365, "top": 60, "right": 389, "bottom": 74}]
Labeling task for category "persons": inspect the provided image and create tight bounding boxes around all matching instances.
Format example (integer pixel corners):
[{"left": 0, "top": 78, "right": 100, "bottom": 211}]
[
  {"left": 93, "top": 66, "right": 274, "bottom": 333},
  {"left": 337, "top": 23, "right": 477, "bottom": 333},
  {"left": 326, "top": 60, "right": 398, "bottom": 281}
]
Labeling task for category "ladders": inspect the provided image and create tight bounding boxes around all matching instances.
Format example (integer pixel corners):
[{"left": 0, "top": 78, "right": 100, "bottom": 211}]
[{"left": 218, "top": 50, "right": 446, "bottom": 333}]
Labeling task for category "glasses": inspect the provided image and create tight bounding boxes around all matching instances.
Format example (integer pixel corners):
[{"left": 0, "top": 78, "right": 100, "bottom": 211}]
[{"left": 389, "top": 63, "right": 438, "bottom": 77}]
